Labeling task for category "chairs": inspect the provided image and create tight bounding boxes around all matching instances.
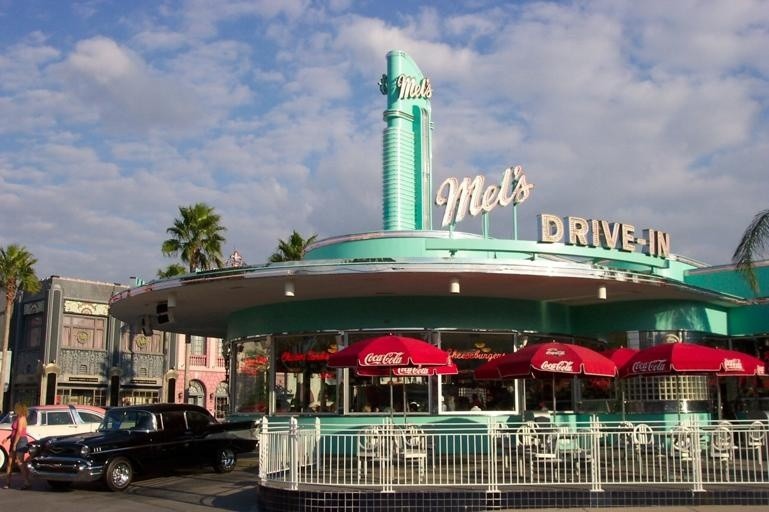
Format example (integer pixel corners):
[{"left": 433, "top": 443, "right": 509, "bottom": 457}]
[
  {"left": 615, "top": 421, "right": 768, "bottom": 470},
  {"left": 356, "top": 423, "right": 438, "bottom": 484},
  {"left": 492, "top": 421, "right": 609, "bottom": 485}
]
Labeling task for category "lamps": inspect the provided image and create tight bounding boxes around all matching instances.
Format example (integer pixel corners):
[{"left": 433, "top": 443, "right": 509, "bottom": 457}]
[
  {"left": 285, "top": 282, "right": 295, "bottom": 297},
  {"left": 448, "top": 279, "right": 460, "bottom": 293},
  {"left": 597, "top": 286, "right": 606, "bottom": 300}
]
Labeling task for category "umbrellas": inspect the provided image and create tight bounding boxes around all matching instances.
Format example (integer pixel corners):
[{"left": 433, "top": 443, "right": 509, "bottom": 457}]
[
  {"left": 351, "top": 363, "right": 460, "bottom": 411},
  {"left": 473, "top": 339, "right": 618, "bottom": 423},
  {"left": 602, "top": 343, "right": 641, "bottom": 422},
  {"left": 616, "top": 340, "right": 768, "bottom": 419},
  {"left": 326, "top": 334, "right": 455, "bottom": 410}
]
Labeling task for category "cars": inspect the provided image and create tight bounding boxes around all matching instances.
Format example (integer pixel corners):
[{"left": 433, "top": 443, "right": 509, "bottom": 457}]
[
  {"left": 25, "top": 404, "right": 258, "bottom": 490},
  {"left": 0, "top": 403, "right": 138, "bottom": 471}
]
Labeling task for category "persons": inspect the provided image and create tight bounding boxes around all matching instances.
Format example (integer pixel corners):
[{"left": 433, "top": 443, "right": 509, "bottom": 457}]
[{"left": 1, "top": 402, "right": 32, "bottom": 490}]
